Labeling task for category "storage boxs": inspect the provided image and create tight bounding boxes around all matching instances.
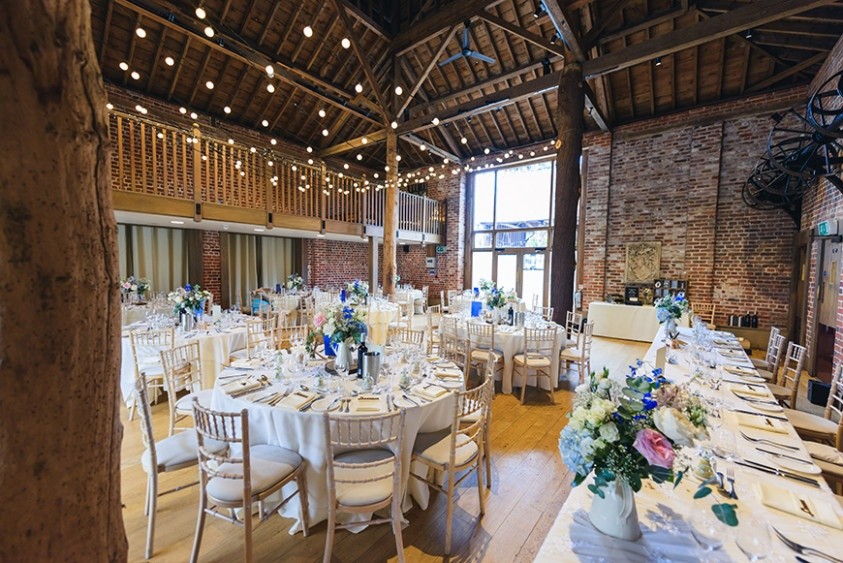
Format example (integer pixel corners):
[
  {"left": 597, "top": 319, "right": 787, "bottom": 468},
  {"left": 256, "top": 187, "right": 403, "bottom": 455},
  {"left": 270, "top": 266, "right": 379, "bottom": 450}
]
[{"left": 808, "top": 379, "right": 839, "bottom": 409}]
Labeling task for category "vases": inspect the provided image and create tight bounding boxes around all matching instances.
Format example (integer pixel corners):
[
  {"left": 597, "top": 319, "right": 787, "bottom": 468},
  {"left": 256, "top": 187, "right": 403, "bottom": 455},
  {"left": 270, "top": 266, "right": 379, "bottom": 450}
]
[
  {"left": 588, "top": 469, "right": 644, "bottom": 541},
  {"left": 334, "top": 339, "right": 354, "bottom": 370},
  {"left": 667, "top": 318, "right": 678, "bottom": 332},
  {"left": 181, "top": 313, "right": 193, "bottom": 332},
  {"left": 484, "top": 289, "right": 490, "bottom": 302}
]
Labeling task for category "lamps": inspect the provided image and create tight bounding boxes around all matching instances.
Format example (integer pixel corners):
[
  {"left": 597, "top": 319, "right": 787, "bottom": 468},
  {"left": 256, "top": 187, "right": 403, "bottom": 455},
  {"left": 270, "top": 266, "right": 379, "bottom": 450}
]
[
  {"left": 131, "top": 1, "right": 502, "bottom": 129},
  {"left": 655, "top": 57, "right": 662, "bottom": 65},
  {"left": 588, "top": 107, "right": 594, "bottom": 116},
  {"left": 746, "top": 29, "right": 753, "bottom": 39},
  {"left": 533, "top": 0, "right": 561, "bottom": 46}
]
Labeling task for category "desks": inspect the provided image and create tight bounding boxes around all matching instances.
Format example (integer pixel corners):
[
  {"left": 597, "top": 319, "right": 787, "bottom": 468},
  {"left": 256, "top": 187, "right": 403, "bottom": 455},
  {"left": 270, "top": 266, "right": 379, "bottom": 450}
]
[{"left": 587, "top": 300, "right": 691, "bottom": 343}]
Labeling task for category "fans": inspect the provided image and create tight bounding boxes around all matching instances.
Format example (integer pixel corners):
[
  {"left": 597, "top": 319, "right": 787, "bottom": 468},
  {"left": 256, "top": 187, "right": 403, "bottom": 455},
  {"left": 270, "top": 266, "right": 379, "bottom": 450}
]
[{"left": 438, "top": 18, "right": 496, "bottom": 67}]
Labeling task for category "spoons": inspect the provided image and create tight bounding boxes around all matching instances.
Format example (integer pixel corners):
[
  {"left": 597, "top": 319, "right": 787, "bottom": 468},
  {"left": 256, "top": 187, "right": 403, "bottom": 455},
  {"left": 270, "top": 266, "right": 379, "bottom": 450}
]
[
  {"left": 403, "top": 395, "right": 421, "bottom": 410},
  {"left": 300, "top": 385, "right": 321, "bottom": 394},
  {"left": 261, "top": 374, "right": 272, "bottom": 386},
  {"left": 339, "top": 399, "right": 351, "bottom": 413}
]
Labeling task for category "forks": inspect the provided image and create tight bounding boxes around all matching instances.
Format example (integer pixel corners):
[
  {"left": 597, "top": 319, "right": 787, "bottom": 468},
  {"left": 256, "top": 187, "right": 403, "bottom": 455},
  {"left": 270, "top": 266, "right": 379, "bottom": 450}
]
[
  {"left": 769, "top": 523, "right": 843, "bottom": 563},
  {"left": 738, "top": 429, "right": 800, "bottom": 452},
  {"left": 270, "top": 386, "right": 292, "bottom": 407}
]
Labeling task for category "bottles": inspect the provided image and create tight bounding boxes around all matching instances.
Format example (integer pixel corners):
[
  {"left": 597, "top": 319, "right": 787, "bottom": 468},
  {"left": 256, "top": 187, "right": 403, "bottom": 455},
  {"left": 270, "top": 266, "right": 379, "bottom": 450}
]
[
  {"left": 357, "top": 332, "right": 367, "bottom": 378},
  {"left": 508, "top": 304, "right": 514, "bottom": 327}
]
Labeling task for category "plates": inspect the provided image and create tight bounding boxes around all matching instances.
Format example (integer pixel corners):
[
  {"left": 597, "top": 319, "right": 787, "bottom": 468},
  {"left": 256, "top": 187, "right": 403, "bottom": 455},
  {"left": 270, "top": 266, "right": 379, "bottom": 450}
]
[
  {"left": 713, "top": 328, "right": 765, "bottom": 383},
  {"left": 393, "top": 394, "right": 421, "bottom": 408},
  {"left": 759, "top": 444, "right": 822, "bottom": 476},
  {"left": 312, "top": 398, "right": 340, "bottom": 411},
  {"left": 746, "top": 396, "right": 782, "bottom": 412},
  {"left": 436, "top": 363, "right": 455, "bottom": 368},
  {"left": 440, "top": 378, "right": 462, "bottom": 389}
]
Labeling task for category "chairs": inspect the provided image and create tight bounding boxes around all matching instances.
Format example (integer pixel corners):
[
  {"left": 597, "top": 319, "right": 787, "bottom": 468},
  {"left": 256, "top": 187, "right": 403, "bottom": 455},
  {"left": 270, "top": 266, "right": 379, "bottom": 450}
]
[
  {"left": 125, "top": 288, "right": 596, "bottom": 563},
  {"left": 744, "top": 326, "right": 843, "bottom": 511}
]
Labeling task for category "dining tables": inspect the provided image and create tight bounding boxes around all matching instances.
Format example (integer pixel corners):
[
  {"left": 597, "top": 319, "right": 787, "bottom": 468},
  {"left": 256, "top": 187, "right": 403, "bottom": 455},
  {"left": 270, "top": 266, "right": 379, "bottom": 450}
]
[
  {"left": 120, "top": 313, "right": 269, "bottom": 410},
  {"left": 258, "top": 288, "right": 331, "bottom": 331},
  {"left": 536, "top": 324, "right": 843, "bottom": 563},
  {"left": 440, "top": 305, "right": 567, "bottom": 394},
  {"left": 318, "top": 295, "right": 402, "bottom": 345},
  {"left": 377, "top": 288, "right": 425, "bottom": 315},
  {"left": 121, "top": 298, "right": 203, "bottom": 327},
  {"left": 452, "top": 291, "right": 525, "bottom": 317},
  {"left": 209, "top": 342, "right": 467, "bottom": 536}
]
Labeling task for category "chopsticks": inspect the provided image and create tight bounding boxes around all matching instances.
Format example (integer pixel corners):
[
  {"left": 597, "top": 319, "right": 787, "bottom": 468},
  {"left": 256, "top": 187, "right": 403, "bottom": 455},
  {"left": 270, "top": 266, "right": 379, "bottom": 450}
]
[
  {"left": 735, "top": 456, "right": 821, "bottom": 487},
  {"left": 722, "top": 378, "right": 766, "bottom": 388},
  {"left": 736, "top": 407, "right": 788, "bottom": 423}
]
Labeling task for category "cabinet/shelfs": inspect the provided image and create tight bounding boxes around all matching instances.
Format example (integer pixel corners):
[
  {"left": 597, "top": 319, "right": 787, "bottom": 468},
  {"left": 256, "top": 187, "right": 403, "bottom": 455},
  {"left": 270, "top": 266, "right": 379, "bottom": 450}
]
[{"left": 691, "top": 302, "right": 716, "bottom": 324}]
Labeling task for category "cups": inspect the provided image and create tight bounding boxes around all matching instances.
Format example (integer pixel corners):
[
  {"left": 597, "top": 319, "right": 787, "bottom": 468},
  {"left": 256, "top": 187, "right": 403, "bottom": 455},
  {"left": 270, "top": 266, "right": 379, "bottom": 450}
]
[
  {"left": 362, "top": 351, "right": 380, "bottom": 387},
  {"left": 729, "top": 314, "right": 757, "bottom": 328},
  {"left": 516, "top": 310, "right": 524, "bottom": 329}
]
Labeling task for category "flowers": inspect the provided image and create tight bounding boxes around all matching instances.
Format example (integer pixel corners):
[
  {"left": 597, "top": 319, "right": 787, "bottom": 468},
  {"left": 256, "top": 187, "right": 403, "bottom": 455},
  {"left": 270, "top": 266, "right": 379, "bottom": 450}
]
[
  {"left": 478, "top": 278, "right": 496, "bottom": 291},
  {"left": 167, "top": 283, "right": 211, "bottom": 322},
  {"left": 484, "top": 286, "right": 508, "bottom": 310},
  {"left": 314, "top": 288, "right": 371, "bottom": 344},
  {"left": 286, "top": 272, "right": 304, "bottom": 290},
  {"left": 121, "top": 276, "right": 152, "bottom": 303},
  {"left": 653, "top": 288, "right": 691, "bottom": 326},
  {"left": 346, "top": 277, "right": 370, "bottom": 306},
  {"left": 557, "top": 358, "right": 739, "bottom": 527}
]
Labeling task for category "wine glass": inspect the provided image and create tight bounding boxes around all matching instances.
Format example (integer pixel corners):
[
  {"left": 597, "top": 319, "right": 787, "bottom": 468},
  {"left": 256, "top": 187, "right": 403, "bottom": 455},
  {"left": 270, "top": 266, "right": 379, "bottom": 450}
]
[
  {"left": 685, "top": 313, "right": 731, "bottom": 563},
  {"left": 735, "top": 518, "right": 773, "bottom": 563}
]
